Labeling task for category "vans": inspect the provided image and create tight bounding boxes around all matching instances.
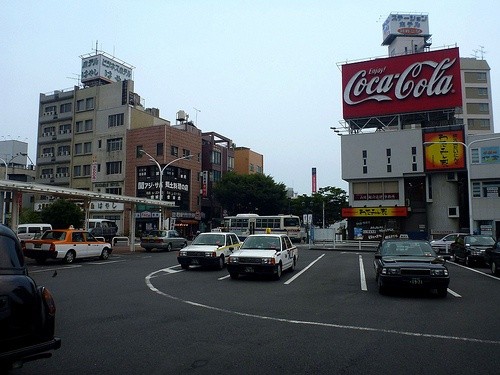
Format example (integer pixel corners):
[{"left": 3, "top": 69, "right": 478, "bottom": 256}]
[
  {"left": 15, "top": 222, "right": 53, "bottom": 242},
  {"left": 84, "top": 218, "right": 119, "bottom": 232},
  {"left": 86, "top": 226, "right": 116, "bottom": 241}
]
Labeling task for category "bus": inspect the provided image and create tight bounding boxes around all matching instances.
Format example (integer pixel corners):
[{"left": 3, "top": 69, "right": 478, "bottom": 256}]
[{"left": 224, "top": 213, "right": 301, "bottom": 241}]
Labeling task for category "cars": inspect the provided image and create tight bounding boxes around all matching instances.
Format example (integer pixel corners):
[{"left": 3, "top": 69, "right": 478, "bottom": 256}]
[
  {"left": 21, "top": 225, "right": 113, "bottom": 263},
  {"left": 0, "top": 222, "right": 61, "bottom": 375},
  {"left": 484, "top": 239, "right": 499, "bottom": 276},
  {"left": 140, "top": 229, "right": 188, "bottom": 252},
  {"left": 176, "top": 232, "right": 243, "bottom": 270},
  {"left": 373, "top": 239, "right": 451, "bottom": 297},
  {"left": 450, "top": 234, "right": 497, "bottom": 266},
  {"left": 227, "top": 233, "right": 299, "bottom": 280},
  {"left": 429, "top": 232, "right": 469, "bottom": 253}
]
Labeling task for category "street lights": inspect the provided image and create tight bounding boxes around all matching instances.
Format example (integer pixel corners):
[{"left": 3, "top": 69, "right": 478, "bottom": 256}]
[
  {"left": 138, "top": 147, "right": 193, "bottom": 230},
  {"left": 423, "top": 137, "right": 500, "bottom": 234},
  {"left": 1, "top": 152, "right": 29, "bottom": 224}
]
